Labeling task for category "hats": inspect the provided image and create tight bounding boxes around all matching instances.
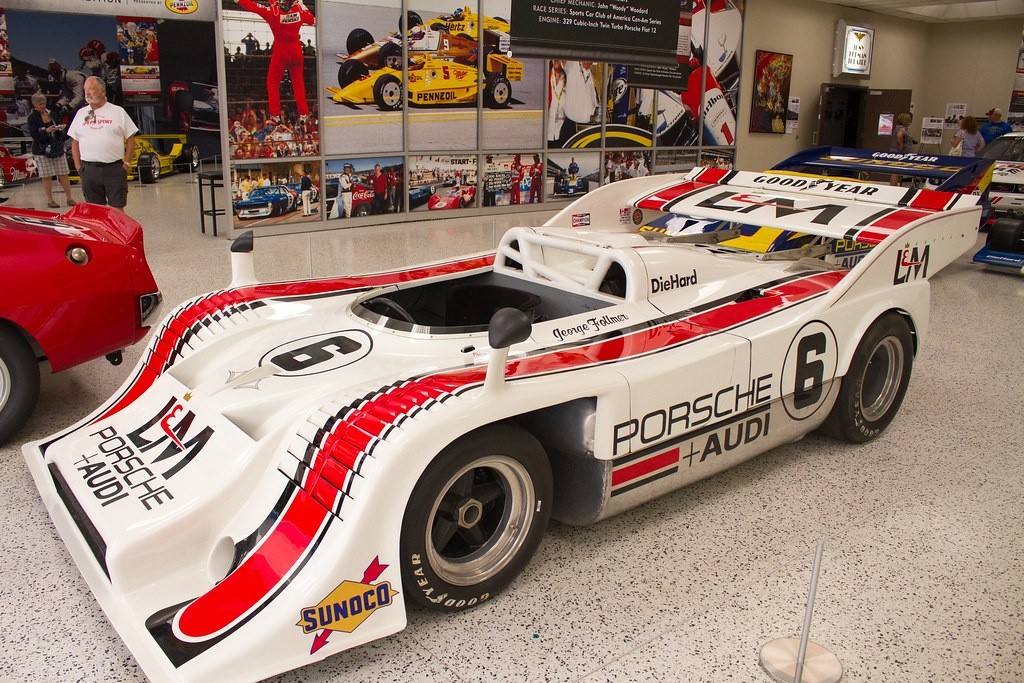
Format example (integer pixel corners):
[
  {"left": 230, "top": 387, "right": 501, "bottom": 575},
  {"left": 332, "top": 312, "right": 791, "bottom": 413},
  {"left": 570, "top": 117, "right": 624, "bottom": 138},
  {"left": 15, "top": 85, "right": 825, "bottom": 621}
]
[{"left": 985, "top": 108, "right": 1002, "bottom": 116}]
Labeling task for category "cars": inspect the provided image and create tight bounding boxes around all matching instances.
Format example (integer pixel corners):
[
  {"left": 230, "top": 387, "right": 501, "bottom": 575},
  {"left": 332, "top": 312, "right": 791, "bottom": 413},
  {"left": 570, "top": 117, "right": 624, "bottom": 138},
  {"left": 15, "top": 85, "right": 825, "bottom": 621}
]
[
  {"left": 325, "top": 176, "right": 381, "bottom": 217},
  {"left": 429, "top": 185, "right": 476, "bottom": 210},
  {"left": 973, "top": 131, "right": 1024, "bottom": 217},
  {"left": 1, "top": 203, "right": 164, "bottom": 446},
  {"left": 287, "top": 182, "right": 316, "bottom": 205},
  {"left": 408, "top": 185, "right": 437, "bottom": 210},
  {"left": 234, "top": 185, "right": 298, "bottom": 220},
  {"left": 22, "top": 142, "right": 984, "bottom": 683}
]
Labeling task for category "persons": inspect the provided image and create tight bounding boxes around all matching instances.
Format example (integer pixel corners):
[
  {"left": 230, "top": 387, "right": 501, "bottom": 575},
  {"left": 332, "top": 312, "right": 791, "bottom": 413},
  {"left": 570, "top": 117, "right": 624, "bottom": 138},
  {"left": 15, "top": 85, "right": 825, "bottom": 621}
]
[
  {"left": 27, "top": 89, "right": 77, "bottom": 208},
  {"left": 606, "top": 151, "right": 651, "bottom": 187},
  {"left": 506, "top": 151, "right": 525, "bottom": 205},
  {"left": 569, "top": 154, "right": 579, "bottom": 184},
  {"left": 948, "top": 116, "right": 985, "bottom": 158},
  {"left": 409, "top": 164, "right": 470, "bottom": 189},
  {"left": 979, "top": 107, "right": 1012, "bottom": 145},
  {"left": 890, "top": 111, "right": 913, "bottom": 186},
  {"left": 118, "top": 19, "right": 158, "bottom": 65},
  {"left": 228, "top": 102, "right": 319, "bottom": 156},
  {"left": 232, "top": 0, "right": 316, "bottom": 126},
  {"left": 338, "top": 160, "right": 355, "bottom": 218},
  {"left": 552, "top": 167, "right": 564, "bottom": 195},
  {"left": 359, "top": 162, "right": 404, "bottom": 213},
  {"left": 7, "top": 39, "right": 121, "bottom": 156},
  {"left": 67, "top": 76, "right": 139, "bottom": 213},
  {"left": 527, "top": 152, "right": 543, "bottom": 204},
  {"left": 548, "top": 59, "right": 598, "bottom": 146},
  {"left": 639, "top": 0, "right": 745, "bottom": 144},
  {"left": 232, "top": 170, "right": 317, "bottom": 217}
]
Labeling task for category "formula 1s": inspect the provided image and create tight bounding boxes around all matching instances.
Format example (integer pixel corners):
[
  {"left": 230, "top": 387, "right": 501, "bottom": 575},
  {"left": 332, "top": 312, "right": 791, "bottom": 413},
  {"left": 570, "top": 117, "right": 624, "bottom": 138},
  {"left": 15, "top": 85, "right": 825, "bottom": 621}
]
[
  {"left": 59, "top": 134, "right": 199, "bottom": 185},
  {"left": 334, "top": 6, "right": 510, "bottom": 66},
  {"left": 324, "top": 53, "right": 524, "bottom": 112}
]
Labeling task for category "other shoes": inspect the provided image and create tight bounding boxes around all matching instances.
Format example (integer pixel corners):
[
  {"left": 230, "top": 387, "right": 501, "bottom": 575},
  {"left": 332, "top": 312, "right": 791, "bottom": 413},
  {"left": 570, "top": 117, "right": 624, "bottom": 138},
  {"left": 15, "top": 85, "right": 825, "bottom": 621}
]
[
  {"left": 67, "top": 198, "right": 77, "bottom": 206},
  {"left": 47, "top": 200, "right": 60, "bottom": 208}
]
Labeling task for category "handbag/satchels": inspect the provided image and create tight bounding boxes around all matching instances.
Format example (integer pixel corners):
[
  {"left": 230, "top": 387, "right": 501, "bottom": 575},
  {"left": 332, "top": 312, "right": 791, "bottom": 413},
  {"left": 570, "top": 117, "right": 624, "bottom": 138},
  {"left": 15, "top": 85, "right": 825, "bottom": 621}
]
[{"left": 949, "top": 139, "right": 963, "bottom": 156}]
